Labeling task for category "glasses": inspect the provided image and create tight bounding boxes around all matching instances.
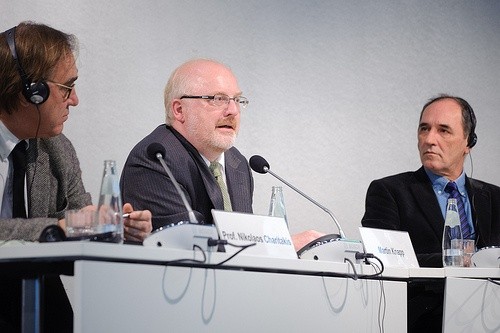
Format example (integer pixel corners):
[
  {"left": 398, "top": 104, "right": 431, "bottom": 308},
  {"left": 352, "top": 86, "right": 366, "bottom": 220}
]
[
  {"left": 180, "top": 93, "right": 250, "bottom": 111},
  {"left": 49, "top": 82, "right": 76, "bottom": 100}
]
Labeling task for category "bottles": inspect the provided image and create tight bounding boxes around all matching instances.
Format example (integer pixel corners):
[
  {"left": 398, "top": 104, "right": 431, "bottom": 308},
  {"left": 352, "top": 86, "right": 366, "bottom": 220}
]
[
  {"left": 442, "top": 199, "right": 463, "bottom": 268},
  {"left": 93, "top": 160, "right": 124, "bottom": 243},
  {"left": 269, "top": 186, "right": 288, "bottom": 230}
]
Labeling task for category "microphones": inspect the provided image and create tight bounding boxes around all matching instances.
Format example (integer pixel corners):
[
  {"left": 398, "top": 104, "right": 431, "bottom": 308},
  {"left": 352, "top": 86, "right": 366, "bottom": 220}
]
[
  {"left": 249, "top": 155, "right": 366, "bottom": 265},
  {"left": 145, "top": 142, "right": 221, "bottom": 251}
]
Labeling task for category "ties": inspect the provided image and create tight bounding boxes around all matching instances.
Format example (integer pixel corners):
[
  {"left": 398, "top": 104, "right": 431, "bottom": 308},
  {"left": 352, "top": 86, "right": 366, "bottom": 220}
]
[
  {"left": 445, "top": 181, "right": 470, "bottom": 248},
  {"left": 11, "top": 141, "right": 27, "bottom": 218},
  {"left": 210, "top": 164, "right": 233, "bottom": 212}
]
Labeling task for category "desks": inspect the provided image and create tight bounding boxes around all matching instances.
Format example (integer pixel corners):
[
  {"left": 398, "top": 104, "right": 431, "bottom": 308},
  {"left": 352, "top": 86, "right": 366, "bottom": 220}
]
[{"left": 0, "top": 241, "right": 500, "bottom": 333}]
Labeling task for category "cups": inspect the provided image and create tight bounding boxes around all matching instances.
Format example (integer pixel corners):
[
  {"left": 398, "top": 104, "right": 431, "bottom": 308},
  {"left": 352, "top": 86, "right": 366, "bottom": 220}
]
[
  {"left": 451, "top": 239, "right": 475, "bottom": 268},
  {"left": 65, "top": 210, "right": 94, "bottom": 241}
]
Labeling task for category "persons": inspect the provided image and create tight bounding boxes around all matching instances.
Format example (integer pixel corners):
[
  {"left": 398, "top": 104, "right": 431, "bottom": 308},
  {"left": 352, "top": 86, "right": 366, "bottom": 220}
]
[
  {"left": 119, "top": 59, "right": 327, "bottom": 254},
  {"left": 361, "top": 94, "right": 500, "bottom": 333},
  {"left": 0, "top": 21, "right": 152, "bottom": 333}
]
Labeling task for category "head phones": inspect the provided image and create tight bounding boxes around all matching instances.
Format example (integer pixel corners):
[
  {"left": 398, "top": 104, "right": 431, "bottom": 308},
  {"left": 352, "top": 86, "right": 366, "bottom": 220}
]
[
  {"left": 5, "top": 25, "right": 50, "bottom": 104},
  {"left": 457, "top": 98, "right": 477, "bottom": 148}
]
